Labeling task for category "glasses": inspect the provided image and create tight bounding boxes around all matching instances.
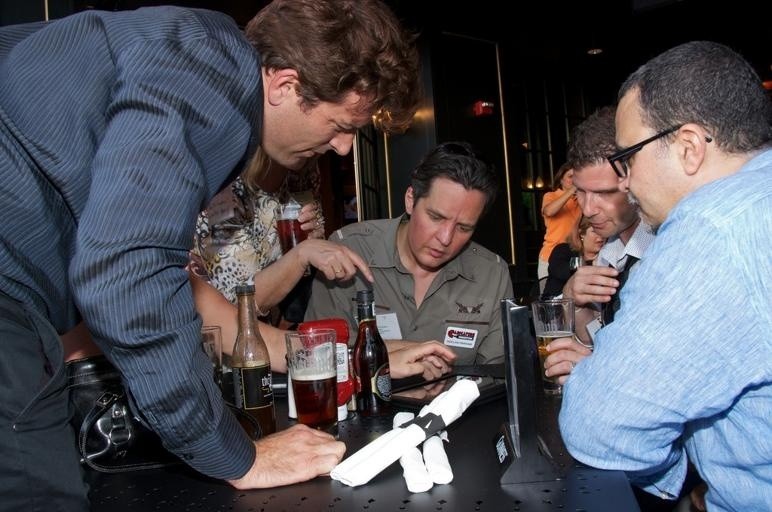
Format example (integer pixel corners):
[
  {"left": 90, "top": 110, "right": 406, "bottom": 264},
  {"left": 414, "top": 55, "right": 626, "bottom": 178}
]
[{"left": 606, "top": 123, "right": 711, "bottom": 178}]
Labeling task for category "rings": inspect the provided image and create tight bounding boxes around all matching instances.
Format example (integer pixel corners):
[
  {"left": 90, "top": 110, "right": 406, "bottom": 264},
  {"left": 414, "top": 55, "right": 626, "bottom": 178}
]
[
  {"left": 334, "top": 268, "right": 344, "bottom": 274},
  {"left": 570, "top": 361, "right": 577, "bottom": 369},
  {"left": 312, "top": 200, "right": 324, "bottom": 229}
]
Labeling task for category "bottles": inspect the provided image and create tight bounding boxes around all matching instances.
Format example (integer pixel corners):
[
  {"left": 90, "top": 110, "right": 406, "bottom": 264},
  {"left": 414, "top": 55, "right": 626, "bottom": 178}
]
[
  {"left": 351, "top": 289, "right": 396, "bottom": 418},
  {"left": 230, "top": 283, "right": 276, "bottom": 440}
]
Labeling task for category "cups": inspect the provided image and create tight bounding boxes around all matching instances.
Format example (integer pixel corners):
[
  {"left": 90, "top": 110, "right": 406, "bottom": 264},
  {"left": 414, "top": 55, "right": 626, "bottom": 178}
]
[
  {"left": 272, "top": 203, "right": 313, "bottom": 277},
  {"left": 199, "top": 324, "right": 224, "bottom": 392},
  {"left": 283, "top": 329, "right": 341, "bottom": 440},
  {"left": 530, "top": 297, "right": 577, "bottom": 398}
]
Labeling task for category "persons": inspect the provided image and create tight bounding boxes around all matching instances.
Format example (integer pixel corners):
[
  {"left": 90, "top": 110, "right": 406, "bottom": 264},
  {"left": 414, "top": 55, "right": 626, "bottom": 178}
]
[
  {"left": 303, "top": 141, "right": 515, "bottom": 382},
  {"left": 541, "top": 104, "right": 663, "bottom": 387},
  {"left": 556, "top": 41, "right": 772, "bottom": 512},
  {"left": 392, "top": 379, "right": 448, "bottom": 400},
  {"left": 535, "top": 162, "right": 583, "bottom": 295},
  {"left": 1, "top": 0, "right": 421, "bottom": 511},
  {"left": 180, "top": 145, "right": 376, "bottom": 318},
  {"left": 539, "top": 215, "right": 606, "bottom": 302},
  {"left": 59, "top": 265, "right": 459, "bottom": 380}
]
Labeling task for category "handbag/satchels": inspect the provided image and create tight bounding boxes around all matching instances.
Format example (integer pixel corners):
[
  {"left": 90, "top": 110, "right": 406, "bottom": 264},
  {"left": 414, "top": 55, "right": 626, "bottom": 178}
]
[{"left": 66, "top": 351, "right": 192, "bottom": 474}]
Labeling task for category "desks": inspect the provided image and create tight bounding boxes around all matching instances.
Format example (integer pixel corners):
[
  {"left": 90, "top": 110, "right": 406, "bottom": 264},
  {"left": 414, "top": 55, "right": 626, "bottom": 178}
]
[{"left": 80, "top": 362, "right": 641, "bottom": 511}]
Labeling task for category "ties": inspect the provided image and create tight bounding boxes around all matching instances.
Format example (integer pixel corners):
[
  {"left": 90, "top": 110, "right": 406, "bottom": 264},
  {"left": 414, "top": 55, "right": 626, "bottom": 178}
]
[{"left": 602, "top": 255, "right": 640, "bottom": 325}]
